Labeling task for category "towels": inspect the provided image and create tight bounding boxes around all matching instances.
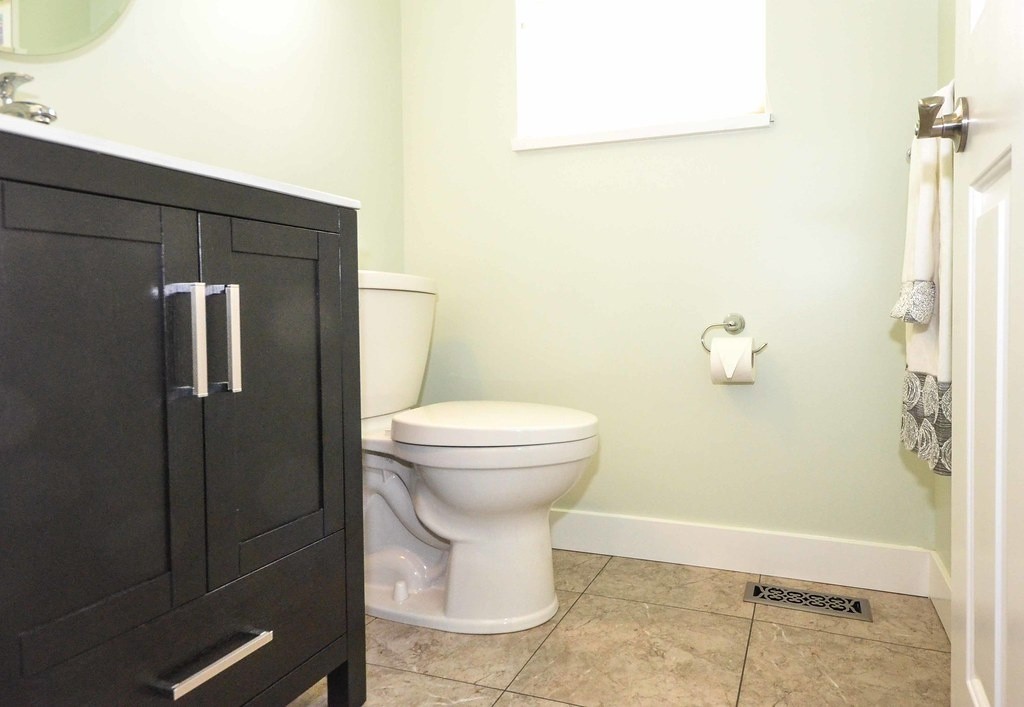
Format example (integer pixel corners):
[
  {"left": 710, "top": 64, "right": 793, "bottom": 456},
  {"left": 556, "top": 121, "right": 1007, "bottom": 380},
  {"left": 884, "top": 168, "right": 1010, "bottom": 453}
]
[{"left": 896, "top": 80, "right": 955, "bottom": 477}]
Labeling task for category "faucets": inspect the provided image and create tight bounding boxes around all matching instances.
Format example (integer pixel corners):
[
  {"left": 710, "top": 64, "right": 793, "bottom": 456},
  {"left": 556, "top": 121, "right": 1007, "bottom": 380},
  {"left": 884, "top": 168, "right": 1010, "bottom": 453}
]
[{"left": 0, "top": 70, "right": 57, "bottom": 125}]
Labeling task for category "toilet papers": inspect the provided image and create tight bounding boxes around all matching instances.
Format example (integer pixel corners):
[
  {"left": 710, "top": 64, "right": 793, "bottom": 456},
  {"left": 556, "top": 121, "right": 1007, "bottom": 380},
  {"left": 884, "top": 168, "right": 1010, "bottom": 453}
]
[{"left": 708, "top": 335, "right": 757, "bottom": 385}]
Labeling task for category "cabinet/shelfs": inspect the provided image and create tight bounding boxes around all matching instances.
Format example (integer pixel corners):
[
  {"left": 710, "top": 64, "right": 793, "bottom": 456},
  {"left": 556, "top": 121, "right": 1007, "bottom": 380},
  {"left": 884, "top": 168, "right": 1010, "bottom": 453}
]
[{"left": 0, "top": 132, "right": 367, "bottom": 707}]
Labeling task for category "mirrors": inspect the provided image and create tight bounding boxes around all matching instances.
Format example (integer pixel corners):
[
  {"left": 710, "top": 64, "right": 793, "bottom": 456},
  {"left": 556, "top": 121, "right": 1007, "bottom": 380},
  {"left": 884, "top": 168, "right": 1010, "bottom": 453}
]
[{"left": 0, "top": 0, "right": 130, "bottom": 56}]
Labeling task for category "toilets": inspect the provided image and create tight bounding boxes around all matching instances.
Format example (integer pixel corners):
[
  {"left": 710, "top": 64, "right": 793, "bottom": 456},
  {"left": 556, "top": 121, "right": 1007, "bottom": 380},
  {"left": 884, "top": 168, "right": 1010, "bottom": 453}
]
[{"left": 356, "top": 268, "right": 602, "bottom": 641}]
